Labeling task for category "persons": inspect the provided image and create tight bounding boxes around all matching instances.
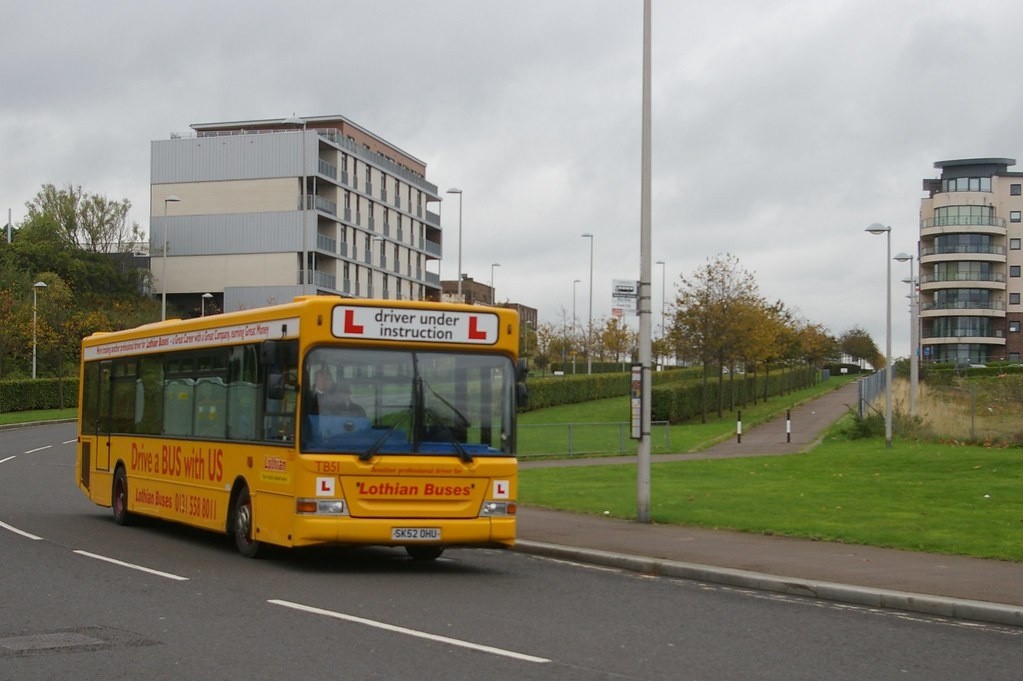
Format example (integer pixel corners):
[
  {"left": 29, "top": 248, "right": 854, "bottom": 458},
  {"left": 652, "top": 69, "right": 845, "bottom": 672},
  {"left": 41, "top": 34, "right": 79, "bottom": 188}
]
[{"left": 310, "top": 369, "right": 366, "bottom": 417}]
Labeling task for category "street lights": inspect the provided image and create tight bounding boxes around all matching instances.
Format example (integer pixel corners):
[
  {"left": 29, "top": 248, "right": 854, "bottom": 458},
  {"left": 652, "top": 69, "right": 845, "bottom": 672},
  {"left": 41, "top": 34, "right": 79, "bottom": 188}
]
[
  {"left": 445, "top": 188, "right": 464, "bottom": 299},
  {"left": 581, "top": 232, "right": 594, "bottom": 374},
  {"left": 864, "top": 222, "right": 894, "bottom": 446},
  {"left": 523, "top": 320, "right": 532, "bottom": 355},
  {"left": 32, "top": 282, "right": 48, "bottom": 380},
  {"left": 655, "top": 260, "right": 666, "bottom": 372},
  {"left": 491, "top": 262, "right": 502, "bottom": 306},
  {"left": 161, "top": 194, "right": 183, "bottom": 321},
  {"left": 572, "top": 279, "right": 582, "bottom": 377},
  {"left": 282, "top": 116, "right": 309, "bottom": 297},
  {"left": 201, "top": 293, "right": 214, "bottom": 318},
  {"left": 892, "top": 252, "right": 918, "bottom": 419}
]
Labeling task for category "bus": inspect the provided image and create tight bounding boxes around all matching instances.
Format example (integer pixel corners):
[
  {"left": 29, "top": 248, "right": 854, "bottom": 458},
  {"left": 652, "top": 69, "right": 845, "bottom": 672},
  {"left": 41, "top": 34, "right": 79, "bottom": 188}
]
[{"left": 74, "top": 295, "right": 528, "bottom": 562}]
